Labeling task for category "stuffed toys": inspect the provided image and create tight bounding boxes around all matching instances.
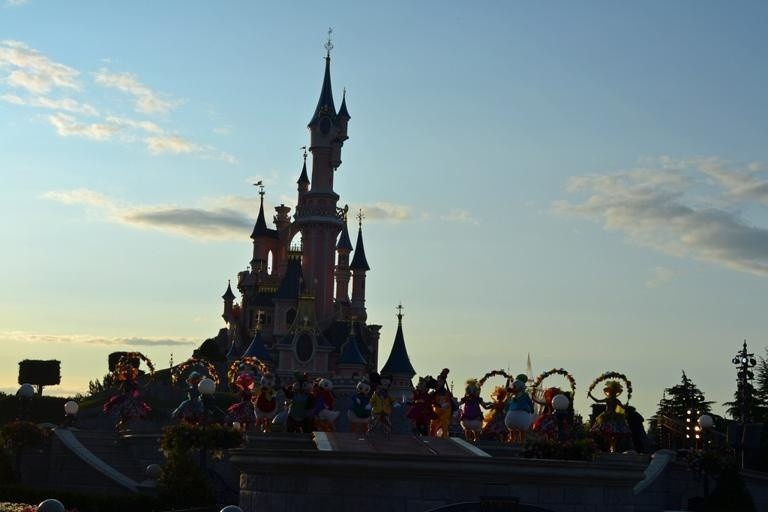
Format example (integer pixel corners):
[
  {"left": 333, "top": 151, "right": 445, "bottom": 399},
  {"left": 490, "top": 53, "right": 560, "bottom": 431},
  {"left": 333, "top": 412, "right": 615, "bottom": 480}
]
[
  {"left": 103, "top": 369, "right": 395, "bottom": 440},
  {"left": 101, "top": 369, "right": 633, "bottom": 454},
  {"left": 406, "top": 375, "right": 633, "bottom": 454}
]
